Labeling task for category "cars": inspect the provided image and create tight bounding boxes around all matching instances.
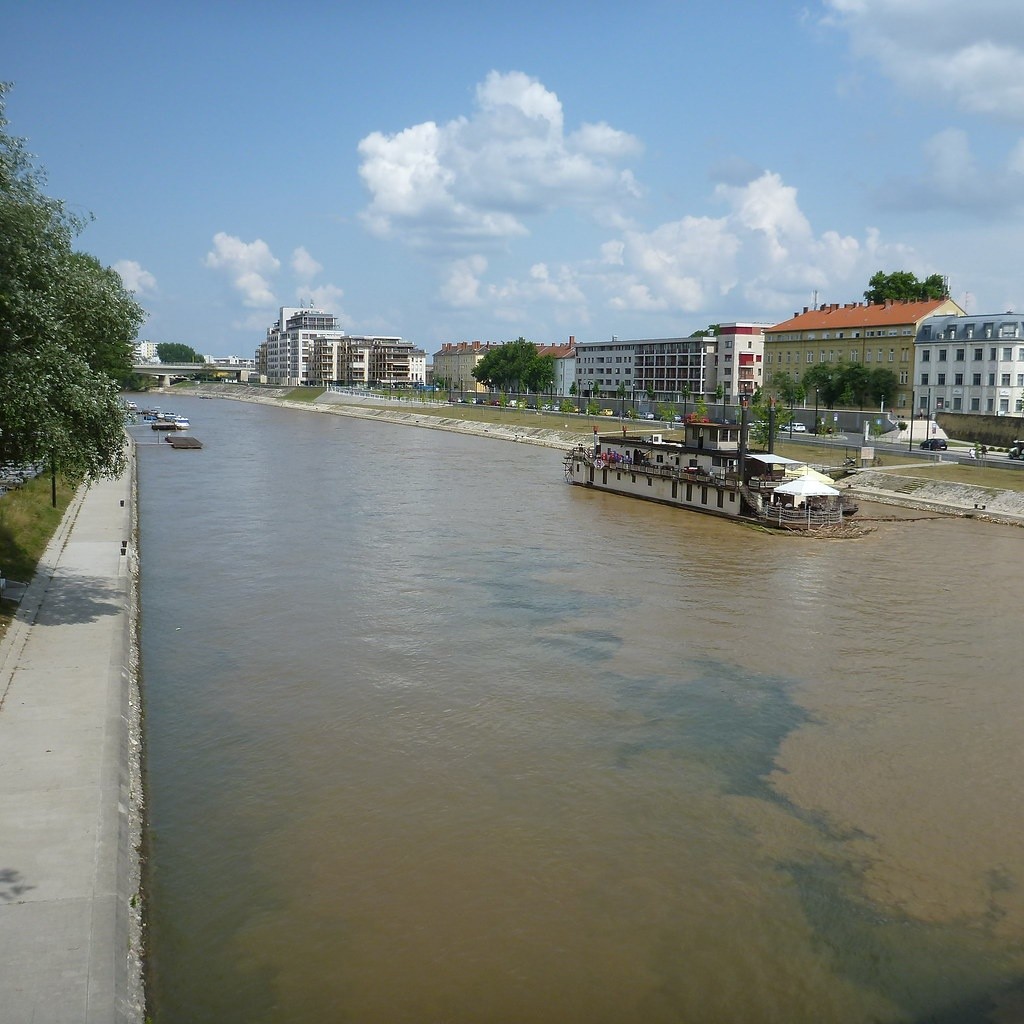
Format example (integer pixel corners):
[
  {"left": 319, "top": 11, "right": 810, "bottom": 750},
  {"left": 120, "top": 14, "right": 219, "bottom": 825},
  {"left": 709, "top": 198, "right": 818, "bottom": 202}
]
[
  {"left": 477, "top": 398, "right": 487, "bottom": 405},
  {"left": 491, "top": 400, "right": 501, "bottom": 407},
  {"left": 447, "top": 397, "right": 477, "bottom": 404},
  {"left": 660, "top": 414, "right": 787, "bottom": 431},
  {"left": 784, "top": 422, "right": 806, "bottom": 433},
  {"left": 626, "top": 410, "right": 654, "bottom": 420},
  {"left": 572, "top": 406, "right": 581, "bottom": 412},
  {"left": 808, "top": 424, "right": 832, "bottom": 434},
  {"left": 506, "top": 399, "right": 560, "bottom": 411}
]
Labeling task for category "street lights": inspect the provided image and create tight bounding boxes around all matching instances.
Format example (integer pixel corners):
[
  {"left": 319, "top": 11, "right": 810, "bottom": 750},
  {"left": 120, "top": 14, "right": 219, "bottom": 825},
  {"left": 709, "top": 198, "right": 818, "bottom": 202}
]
[
  {"left": 461, "top": 379, "right": 463, "bottom": 397},
  {"left": 433, "top": 377, "right": 435, "bottom": 403},
  {"left": 816, "top": 388, "right": 820, "bottom": 433},
  {"left": 588, "top": 382, "right": 590, "bottom": 406},
  {"left": 684, "top": 386, "right": 687, "bottom": 425},
  {"left": 632, "top": 384, "right": 635, "bottom": 412},
  {"left": 745, "top": 383, "right": 747, "bottom": 401},
  {"left": 550, "top": 381, "right": 553, "bottom": 402}
]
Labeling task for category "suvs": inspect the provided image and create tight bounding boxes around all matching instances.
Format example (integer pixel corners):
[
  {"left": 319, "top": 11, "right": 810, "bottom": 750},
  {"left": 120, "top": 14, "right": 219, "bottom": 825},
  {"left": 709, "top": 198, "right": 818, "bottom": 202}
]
[{"left": 600, "top": 408, "right": 612, "bottom": 416}]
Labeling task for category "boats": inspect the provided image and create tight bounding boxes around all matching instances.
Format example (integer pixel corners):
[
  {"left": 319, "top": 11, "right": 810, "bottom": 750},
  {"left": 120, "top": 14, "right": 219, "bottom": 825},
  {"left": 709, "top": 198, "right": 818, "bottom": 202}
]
[
  {"left": 920, "top": 438, "right": 948, "bottom": 451},
  {"left": 563, "top": 398, "right": 859, "bottom": 530},
  {"left": 121, "top": 400, "right": 190, "bottom": 434}
]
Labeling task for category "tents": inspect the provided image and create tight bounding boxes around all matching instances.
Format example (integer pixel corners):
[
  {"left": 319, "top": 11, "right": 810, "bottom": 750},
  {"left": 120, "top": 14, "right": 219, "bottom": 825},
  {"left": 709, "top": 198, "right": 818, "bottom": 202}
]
[{"left": 774, "top": 467, "right": 840, "bottom": 512}]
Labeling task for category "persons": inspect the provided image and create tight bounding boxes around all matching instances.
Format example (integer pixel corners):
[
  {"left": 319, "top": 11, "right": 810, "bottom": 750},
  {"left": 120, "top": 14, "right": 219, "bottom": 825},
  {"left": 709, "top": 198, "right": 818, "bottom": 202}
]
[
  {"left": 626, "top": 409, "right": 632, "bottom": 418},
  {"left": 981, "top": 446, "right": 988, "bottom": 459}
]
